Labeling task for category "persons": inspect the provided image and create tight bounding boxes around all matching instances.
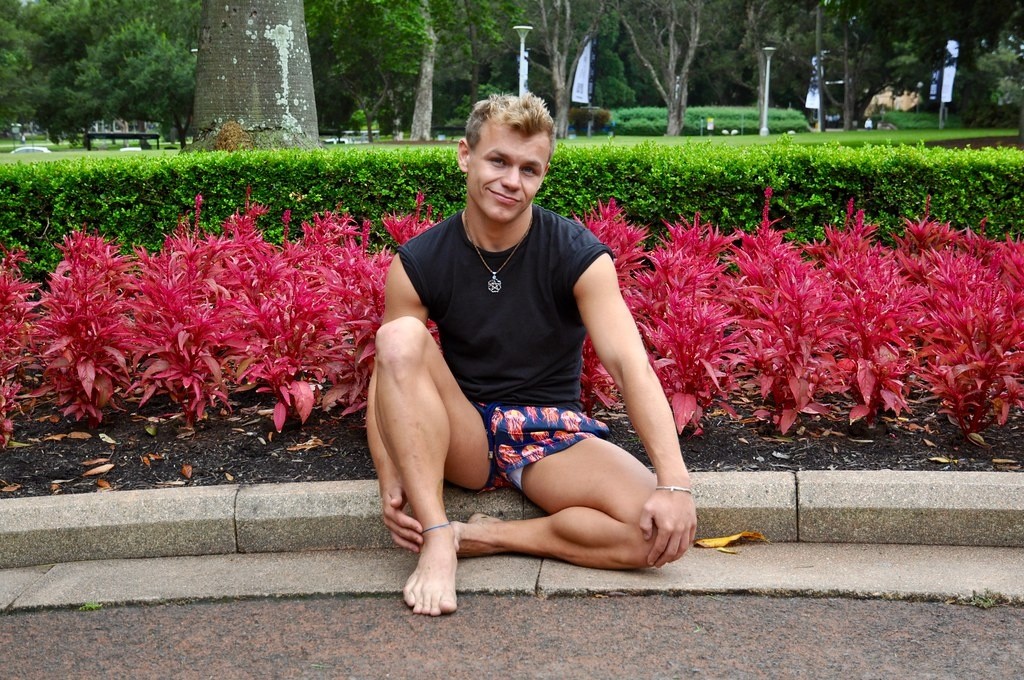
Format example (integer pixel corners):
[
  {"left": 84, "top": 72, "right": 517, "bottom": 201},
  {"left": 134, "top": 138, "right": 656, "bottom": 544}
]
[
  {"left": 865, "top": 118, "right": 872, "bottom": 131},
  {"left": 366, "top": 91, "right": 698, "bottom": 617}
]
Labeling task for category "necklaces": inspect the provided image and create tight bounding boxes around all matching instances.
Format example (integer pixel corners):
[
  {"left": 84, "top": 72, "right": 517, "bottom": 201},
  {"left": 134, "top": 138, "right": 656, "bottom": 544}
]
[{"left": 464, "top": 206, "right": 535, "bottom": 293}]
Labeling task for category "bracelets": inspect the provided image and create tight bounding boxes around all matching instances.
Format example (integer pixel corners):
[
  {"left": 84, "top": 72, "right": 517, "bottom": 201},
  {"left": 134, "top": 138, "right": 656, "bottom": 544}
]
[{"left": 656, "top": 484, "right": 692, "bottom": 497}]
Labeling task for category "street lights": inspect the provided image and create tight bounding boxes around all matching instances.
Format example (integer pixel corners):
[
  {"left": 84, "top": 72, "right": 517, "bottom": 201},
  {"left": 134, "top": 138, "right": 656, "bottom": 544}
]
[
  {"left": 513, "top": 26, "right": 533, "bottom": 98},
  {"left": 759, "top": 46, "right": 777, "bottom": 137}
]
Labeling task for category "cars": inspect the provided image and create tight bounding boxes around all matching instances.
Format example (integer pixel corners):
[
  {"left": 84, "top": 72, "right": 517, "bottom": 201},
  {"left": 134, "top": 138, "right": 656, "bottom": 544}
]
[{"left": 10, "top": 146, "right": 51, "bottom": 154}]
